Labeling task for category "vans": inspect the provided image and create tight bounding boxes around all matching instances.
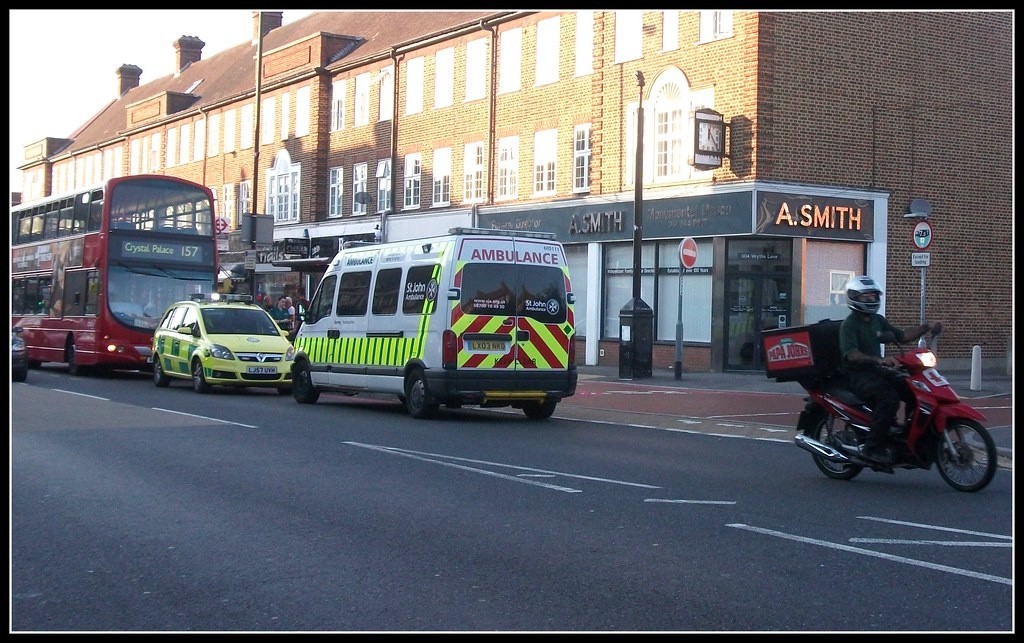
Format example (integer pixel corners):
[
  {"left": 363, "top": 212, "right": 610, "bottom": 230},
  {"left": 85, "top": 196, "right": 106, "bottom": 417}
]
[{"left": 290, "top": 227, "right": 578, "bottom": 419}]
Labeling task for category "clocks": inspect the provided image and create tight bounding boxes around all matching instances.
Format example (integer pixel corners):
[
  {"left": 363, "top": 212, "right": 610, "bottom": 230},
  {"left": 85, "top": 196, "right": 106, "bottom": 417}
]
[{"left": 691, "top": 107, "right": 731, "bottom": 172}]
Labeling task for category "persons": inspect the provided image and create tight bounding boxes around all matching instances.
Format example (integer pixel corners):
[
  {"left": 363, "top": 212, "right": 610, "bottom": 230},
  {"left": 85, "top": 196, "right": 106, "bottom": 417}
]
[
  {"left": 838, "top": 275, "right": 937, "bottom": 466},
  {"left": 289, "top": 286, "right": 310, "bottom": 341},
  {"left": 284, "top": 296, "right": 296, "bottom": 330},
  {"left": 270, "top": 296, "right": 292, "bottom": 341},
  {"left": 262, "top": 296, "right": 274, "bottom": 314}
]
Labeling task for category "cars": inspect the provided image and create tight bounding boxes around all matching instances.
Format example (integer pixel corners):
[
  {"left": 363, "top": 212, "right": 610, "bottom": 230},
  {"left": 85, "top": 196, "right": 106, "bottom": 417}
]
[
  {"left": 151, "top": 292, "right": 296, "bottom": 393},
  {"left": 12, "top": 326, "right": 29, "bottom": 382}
]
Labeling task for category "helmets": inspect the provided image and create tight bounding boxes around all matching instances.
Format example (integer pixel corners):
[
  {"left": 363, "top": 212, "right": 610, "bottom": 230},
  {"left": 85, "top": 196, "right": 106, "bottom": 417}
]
[{"left": 845, "top": 276, "right": 884, "bottom": 316}]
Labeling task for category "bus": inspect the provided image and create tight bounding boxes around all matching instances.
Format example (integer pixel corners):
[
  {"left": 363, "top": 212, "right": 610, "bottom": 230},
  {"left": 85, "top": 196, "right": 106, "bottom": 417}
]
[{"left": 12, "top": 173, "right": 219, "bottom": 377}]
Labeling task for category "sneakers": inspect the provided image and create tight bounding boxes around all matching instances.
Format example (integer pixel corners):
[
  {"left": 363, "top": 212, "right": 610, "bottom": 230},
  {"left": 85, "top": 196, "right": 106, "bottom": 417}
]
[{"left": 858, "top": 441, "right": 893, "bottom": 464}]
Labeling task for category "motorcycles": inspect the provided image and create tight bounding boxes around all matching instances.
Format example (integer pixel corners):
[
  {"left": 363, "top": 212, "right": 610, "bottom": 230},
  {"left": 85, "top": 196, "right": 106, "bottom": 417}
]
[{"left": 794, "top": 320, "right": 999, "bottom": 493}]
